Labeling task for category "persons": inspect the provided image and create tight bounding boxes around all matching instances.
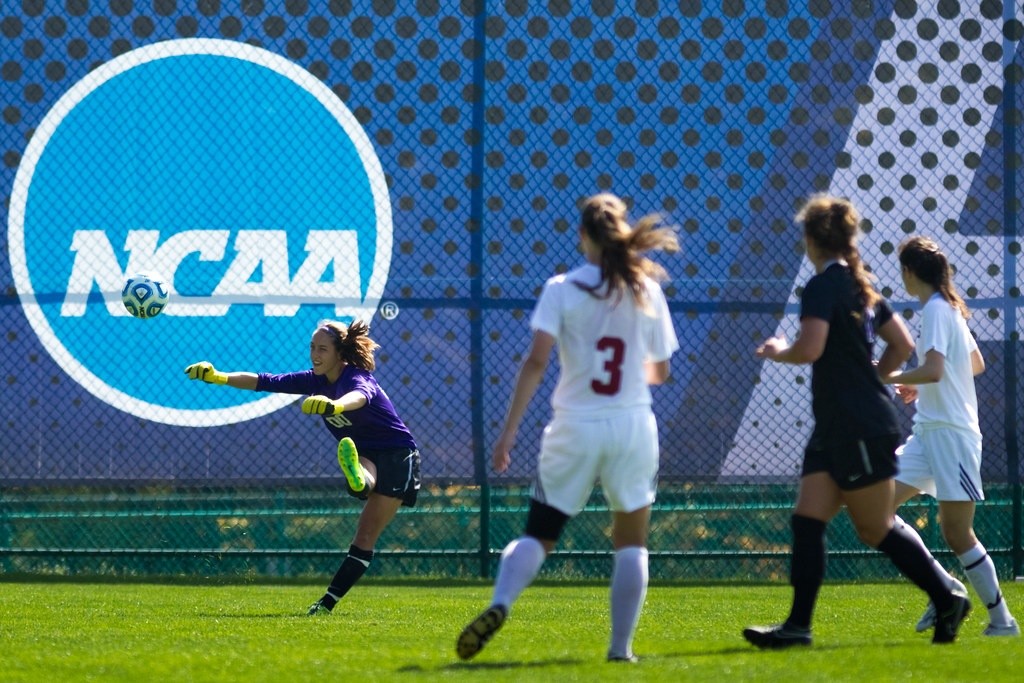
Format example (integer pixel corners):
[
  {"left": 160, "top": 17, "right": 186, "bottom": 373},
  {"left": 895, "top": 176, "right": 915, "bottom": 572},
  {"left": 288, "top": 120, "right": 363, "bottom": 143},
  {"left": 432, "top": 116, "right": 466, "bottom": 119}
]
[
  {"left": 887, "top": 237, "right": 1019, "bottom": 637},
  {"left": 741, "top": 198, "right": 971, "bottom": 649},
  {"left": 184, "top": 319, "right": 420, "bottom": 617},
  {"left": 457, "top": 191, "right": 687, "bottom": 663}
]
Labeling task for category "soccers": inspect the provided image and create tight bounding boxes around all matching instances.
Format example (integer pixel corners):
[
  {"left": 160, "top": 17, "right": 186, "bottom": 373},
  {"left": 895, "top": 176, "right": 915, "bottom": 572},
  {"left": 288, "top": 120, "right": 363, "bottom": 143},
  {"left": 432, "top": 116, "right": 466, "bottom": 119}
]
[{"left": 121, "top": 272, "right": 169, "bottom": 319}]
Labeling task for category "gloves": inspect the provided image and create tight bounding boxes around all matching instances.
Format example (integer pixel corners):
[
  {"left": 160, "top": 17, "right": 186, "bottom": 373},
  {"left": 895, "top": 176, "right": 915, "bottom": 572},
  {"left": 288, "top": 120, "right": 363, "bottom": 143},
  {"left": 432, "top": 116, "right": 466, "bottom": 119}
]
[
  {"left": 301, "top": 394, "right": 344, "bottom": 415},
  {"left": 185, "top": 361, "right": 229, "bottom": 385}
]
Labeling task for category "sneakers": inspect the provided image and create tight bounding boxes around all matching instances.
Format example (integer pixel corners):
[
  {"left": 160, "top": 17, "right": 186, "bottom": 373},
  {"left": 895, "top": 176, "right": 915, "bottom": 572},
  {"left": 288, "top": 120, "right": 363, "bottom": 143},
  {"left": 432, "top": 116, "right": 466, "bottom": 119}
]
[
  {"left": 742, "top": 624, "right": 814, "bottom": 651},
  {"left": 982, "top": 616, "right": 1021, "bottom": 637},
  {"left": 336, "top": 436, "right": 366, "bottom": 492},
  {"left": 608, "top": 650, "right": 639, "bottom": 664},
  {"left": 915, "top": 579, "right": 968, "bottom": 632},
  {"left": 932, "top": 593, "right": 973, "bottom": 644},
  {"left": 456, "top": 604, "right": 506, "bottom": 660},
  {"left": 305, "top": 600, "right": 331, "bottom": 617}
]
